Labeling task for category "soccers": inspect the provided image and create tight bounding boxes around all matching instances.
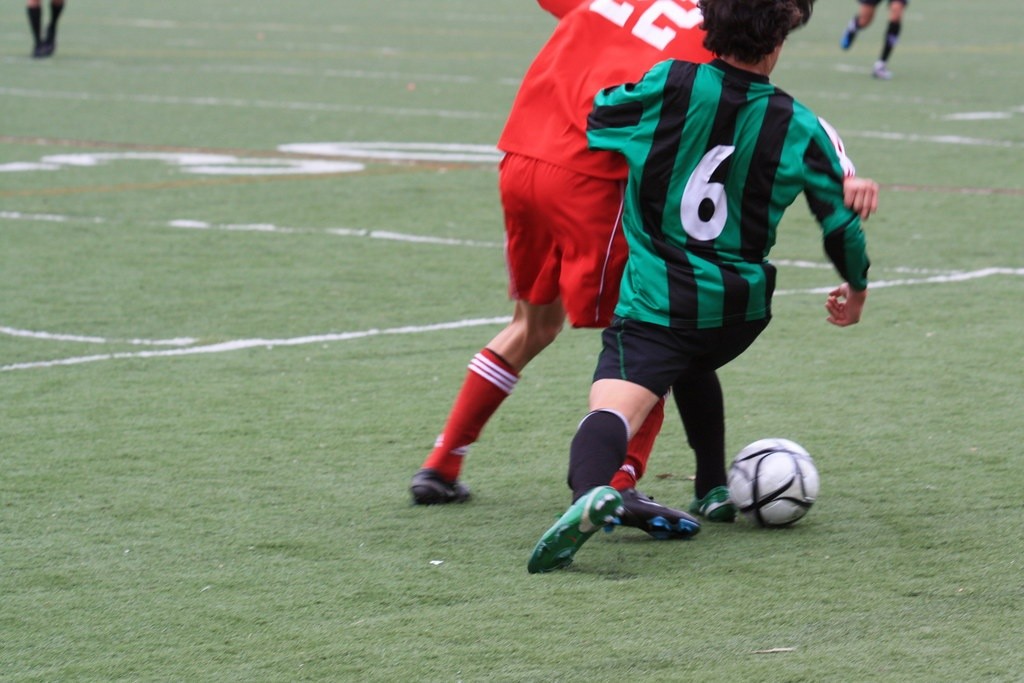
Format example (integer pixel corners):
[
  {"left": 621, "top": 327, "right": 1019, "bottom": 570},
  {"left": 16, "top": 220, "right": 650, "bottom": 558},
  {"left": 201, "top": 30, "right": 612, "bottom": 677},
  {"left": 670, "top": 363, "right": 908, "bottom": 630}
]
[{"left": 729, "top": 437, "right": 819, "bottom": 527}]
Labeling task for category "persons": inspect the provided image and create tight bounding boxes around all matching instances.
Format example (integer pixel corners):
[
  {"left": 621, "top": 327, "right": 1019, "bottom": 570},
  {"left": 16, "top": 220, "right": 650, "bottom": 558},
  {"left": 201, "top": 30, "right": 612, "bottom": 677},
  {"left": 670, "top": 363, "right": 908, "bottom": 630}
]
[
  {"left": 840, "top": 0, "right": 911, "bottom": 81},
  {"left": 26, "top": 0, "right": 65, "bottom": 58},
  {"left": 527, "top": 0, "right": 871, "bottom": 574},
  {"left": 407, "top": 0, "right": 880, "bottom": 541}
]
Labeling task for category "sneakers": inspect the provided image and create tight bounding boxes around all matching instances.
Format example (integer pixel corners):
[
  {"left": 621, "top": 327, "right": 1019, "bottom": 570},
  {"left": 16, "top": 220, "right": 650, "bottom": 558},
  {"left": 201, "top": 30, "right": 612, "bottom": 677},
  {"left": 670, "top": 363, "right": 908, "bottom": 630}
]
[
  {"left": 607, "top": 488, "right": 701, "bottom": 539},
  {"left": 689, "top": 486, "right": 736, "bottom": 520},
  {"left": 410, "top": 469, "right": 471, "bottom": 503},
  {"left": 528, "top": 486, "right": 624, "bottom": 574}
]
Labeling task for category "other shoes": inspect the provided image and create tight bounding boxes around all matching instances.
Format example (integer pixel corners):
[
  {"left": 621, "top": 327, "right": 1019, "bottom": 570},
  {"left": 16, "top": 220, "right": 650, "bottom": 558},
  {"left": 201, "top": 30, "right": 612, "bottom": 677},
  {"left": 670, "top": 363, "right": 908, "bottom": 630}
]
[
  {"left": 34, "top": 42, "right": 54, "bottom": 57},
  {"left": 873, "top": 62, "right": 892, "bottom": 79},
  {"left": 840, "top": 27, "right": 855, "bottom": 48}
]
